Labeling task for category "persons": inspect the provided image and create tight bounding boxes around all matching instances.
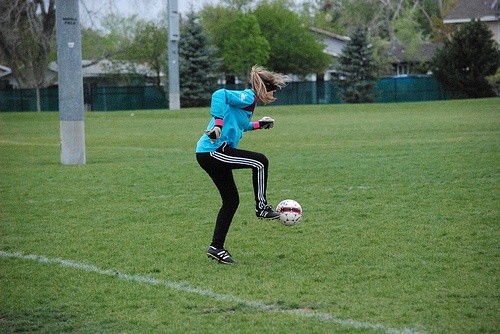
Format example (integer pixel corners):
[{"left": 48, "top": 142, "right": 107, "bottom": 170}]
[{"left": 195, "top": 62, "right": 290, "bottom": 265}]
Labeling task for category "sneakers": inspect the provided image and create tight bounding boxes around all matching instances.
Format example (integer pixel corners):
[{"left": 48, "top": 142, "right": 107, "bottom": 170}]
[
  {"left": 206, "top": 246, "right": 236, "bottom": 264},
  {"left": 255, "top": 204, "right": 281, "bottom": 220}
]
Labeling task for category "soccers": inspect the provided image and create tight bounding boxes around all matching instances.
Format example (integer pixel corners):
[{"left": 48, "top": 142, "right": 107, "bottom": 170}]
[{"left": 275, "top": 199, "right": 303, "bottom": 226}]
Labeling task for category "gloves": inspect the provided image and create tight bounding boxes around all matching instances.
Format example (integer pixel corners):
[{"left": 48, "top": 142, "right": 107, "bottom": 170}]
[
  {"left": 203, "top": 124, "right": 222, "bottom": 141},
  {"left": 258, "top": 116, "right": 275, "bottom": 129}
]
